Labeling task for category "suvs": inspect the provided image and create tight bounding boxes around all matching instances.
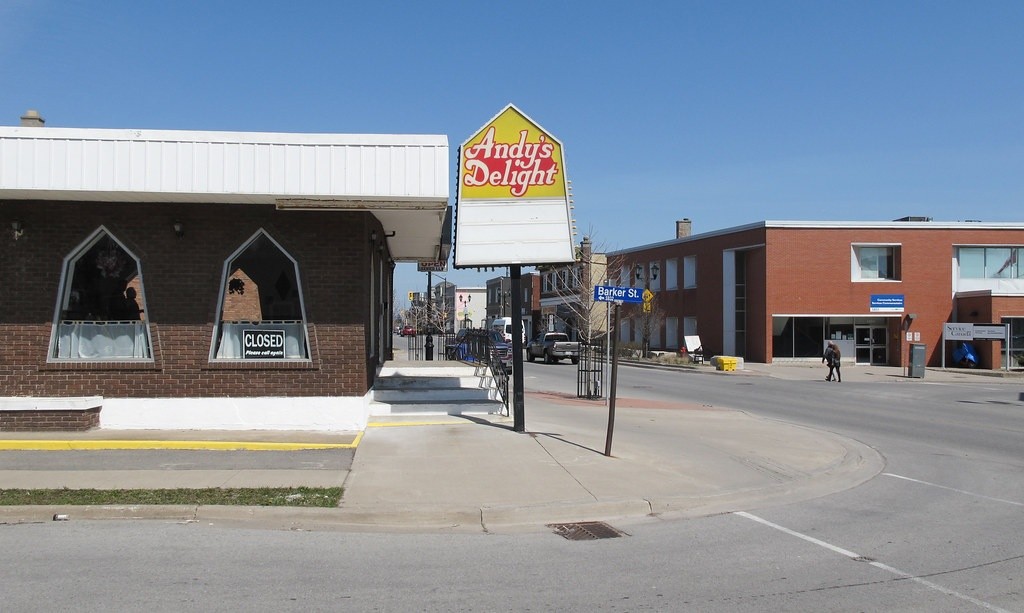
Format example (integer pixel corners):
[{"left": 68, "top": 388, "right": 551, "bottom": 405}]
[
  {"left": 394, "top": 325, "right": 416, "bottom": 337},
  {"left": 455, "top": 329, "right": 513, "bottom": 376}
]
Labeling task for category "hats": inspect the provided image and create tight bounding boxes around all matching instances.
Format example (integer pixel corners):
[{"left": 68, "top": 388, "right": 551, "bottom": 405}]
[{"left": 827, "top": 340, "right": 834, "bottom": 345}]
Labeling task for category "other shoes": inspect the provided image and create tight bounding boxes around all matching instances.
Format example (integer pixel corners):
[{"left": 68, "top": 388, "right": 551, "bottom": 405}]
[
  {"left": 832, "top": 378, "right": 837, "bottom": 382},
  {"left": 824, "top": 375, "right": 831, "bottom": 381},
  {"left": 837, "top": 379, "right": 842, "bottom": 382}
]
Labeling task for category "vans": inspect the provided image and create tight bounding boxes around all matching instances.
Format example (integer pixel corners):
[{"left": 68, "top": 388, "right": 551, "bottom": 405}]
[{"left": 493, "top": 319, "right": 525, "bottom": 346}]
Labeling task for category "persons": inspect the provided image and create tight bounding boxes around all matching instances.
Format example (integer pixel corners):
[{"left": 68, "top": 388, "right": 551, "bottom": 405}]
[
  {"left": 124, "top": 287, "right": 144, "bottom": 320},
  {"left": 821, "top": 341, "right": 842, "bottom": 382}
]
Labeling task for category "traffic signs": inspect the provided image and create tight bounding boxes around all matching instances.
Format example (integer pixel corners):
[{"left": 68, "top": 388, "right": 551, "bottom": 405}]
[{"left": 594, "top": 285, "right": 644, "bottom": 304}]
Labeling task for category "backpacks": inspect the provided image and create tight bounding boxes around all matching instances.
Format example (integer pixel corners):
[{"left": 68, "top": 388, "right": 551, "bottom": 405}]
[{"left": 832, "top": 349, "right": 840, "bottom": 365}]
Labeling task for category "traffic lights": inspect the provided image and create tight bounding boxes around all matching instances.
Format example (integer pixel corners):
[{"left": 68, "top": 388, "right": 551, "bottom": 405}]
[{"left": 408, "top": 292, "right": 413, "bottom": 301}]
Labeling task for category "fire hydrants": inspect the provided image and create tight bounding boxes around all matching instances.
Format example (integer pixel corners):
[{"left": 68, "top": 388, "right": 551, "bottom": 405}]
[{"left": 680, "top": 346, "right": 686, "bottom": 358}]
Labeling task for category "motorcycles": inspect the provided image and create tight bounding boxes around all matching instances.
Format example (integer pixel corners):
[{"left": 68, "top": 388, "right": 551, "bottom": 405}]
[{"left": 953, "top": 342, "right": 976, "bottom": 369}]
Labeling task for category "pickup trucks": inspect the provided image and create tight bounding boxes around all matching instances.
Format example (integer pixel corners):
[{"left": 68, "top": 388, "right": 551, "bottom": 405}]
[{"left": 525, "top": 333, "right": 583, "bottom": 364}]
